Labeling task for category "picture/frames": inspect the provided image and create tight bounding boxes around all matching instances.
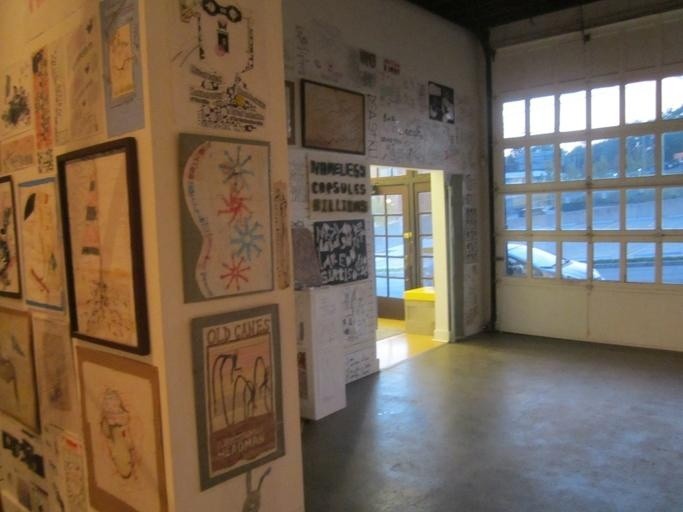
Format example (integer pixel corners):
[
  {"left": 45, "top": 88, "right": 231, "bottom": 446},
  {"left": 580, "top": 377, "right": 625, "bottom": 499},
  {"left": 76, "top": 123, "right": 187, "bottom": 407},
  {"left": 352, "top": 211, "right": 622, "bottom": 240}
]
[
  {"left": 299, "top": 77, "right": 365, "bottom": 157},
  {"left": 55, "top": 137, "right": 151, "bottom": 355}
]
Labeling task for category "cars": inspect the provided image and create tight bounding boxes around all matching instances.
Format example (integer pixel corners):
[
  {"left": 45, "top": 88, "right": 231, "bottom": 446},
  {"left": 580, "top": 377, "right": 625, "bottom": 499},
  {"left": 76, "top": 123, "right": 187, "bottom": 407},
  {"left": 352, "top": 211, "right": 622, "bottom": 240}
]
[{"left": 375, "top": 242, "right": 604, "bottom": 281}]
